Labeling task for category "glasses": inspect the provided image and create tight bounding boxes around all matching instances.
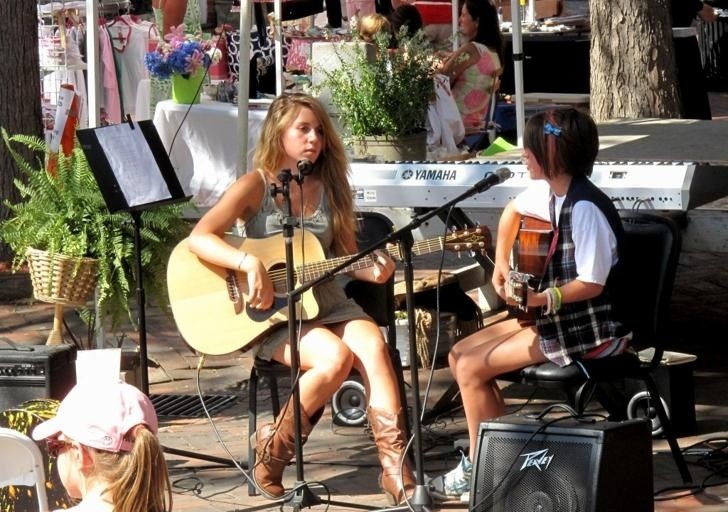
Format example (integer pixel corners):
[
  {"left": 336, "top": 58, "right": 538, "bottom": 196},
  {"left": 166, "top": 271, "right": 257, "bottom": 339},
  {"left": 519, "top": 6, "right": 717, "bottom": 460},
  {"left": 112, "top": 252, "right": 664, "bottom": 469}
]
[{"left": 47, "top": 438, "right": 78, "bottom": 458}]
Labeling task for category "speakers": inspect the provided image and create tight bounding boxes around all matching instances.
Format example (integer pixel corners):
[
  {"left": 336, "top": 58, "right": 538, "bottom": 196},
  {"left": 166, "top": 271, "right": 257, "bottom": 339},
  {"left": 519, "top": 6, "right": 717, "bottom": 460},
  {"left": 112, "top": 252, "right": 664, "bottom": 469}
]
[
  {"left": 332, "top": 376, "right": 367, "bottom": 427},
  {"left": 0, "top": 344, "right": 73, "bottom": 416},
  {"left": 469, "top": 418, "right": 653, "bottom": 512},
  {"left": 624, "top": 368, "right": 696, "bottom": 438}
]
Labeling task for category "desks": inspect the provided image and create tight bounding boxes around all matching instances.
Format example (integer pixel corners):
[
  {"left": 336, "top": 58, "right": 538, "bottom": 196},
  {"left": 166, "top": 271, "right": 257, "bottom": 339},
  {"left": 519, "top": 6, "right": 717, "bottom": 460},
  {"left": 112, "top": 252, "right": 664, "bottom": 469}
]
[{"left": 155, "top": 95, "right": 351, "bottom": 207}]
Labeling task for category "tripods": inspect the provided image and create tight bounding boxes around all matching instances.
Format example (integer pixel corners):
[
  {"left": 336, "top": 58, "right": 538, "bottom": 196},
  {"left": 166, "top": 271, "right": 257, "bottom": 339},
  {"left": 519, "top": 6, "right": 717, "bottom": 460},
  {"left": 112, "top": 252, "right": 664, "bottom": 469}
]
[
  {"left": 370, "top": 240, "right": 469, "bottom": 512},
  {"left": 227, "top": 228, "right": 382, "bottom": 512}
]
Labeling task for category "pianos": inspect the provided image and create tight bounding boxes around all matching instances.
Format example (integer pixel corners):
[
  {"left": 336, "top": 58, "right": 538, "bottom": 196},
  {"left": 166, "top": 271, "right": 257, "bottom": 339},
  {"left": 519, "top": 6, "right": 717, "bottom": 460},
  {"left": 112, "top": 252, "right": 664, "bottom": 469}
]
[{"left": 348, "top": 158, "right": 728, "bottom": 210}]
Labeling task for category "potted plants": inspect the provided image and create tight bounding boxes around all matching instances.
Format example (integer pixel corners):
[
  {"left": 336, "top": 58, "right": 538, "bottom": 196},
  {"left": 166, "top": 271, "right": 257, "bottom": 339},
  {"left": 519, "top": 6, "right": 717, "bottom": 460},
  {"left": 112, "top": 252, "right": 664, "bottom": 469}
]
[{"left": 0, "top": 126, "right": 199, "bottom": 334}]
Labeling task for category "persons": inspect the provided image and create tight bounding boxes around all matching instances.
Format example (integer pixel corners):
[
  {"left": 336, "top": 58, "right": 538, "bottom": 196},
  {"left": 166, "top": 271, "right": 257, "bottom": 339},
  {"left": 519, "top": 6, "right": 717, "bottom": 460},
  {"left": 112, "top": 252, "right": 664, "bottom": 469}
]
[
  {"left": 188, "top": 92, "right": 418, "bottom": 507},
  {"left": 356, "top": 0, "right": 504, "bottom": 135},
  {"left": 666, "top": 0, "right": 716, "bottom": 119},
  {"left": 428, "top": 105, "right": 634, "bottom": 501},
  {"left": 151, "top": 0, "right": 209, "bottom": 117},
  {"left": 32, "top": 380, "right": 173, "bottom": 512}
]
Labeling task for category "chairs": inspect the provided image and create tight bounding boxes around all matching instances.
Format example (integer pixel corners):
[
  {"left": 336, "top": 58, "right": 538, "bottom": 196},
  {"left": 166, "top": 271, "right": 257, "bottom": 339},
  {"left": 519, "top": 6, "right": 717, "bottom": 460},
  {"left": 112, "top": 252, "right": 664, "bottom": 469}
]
[
  {"left": 464, "top": 54, "right": 531, "bottom": 151},
  {"left": 248, "top": 212, "right": 414, "bottom": 496},
  {"left": 488, "top": 210, "right": 694, "bottom": 483},
  {"left": 0, "top": 427, "right": 50, "bottom": 512}
]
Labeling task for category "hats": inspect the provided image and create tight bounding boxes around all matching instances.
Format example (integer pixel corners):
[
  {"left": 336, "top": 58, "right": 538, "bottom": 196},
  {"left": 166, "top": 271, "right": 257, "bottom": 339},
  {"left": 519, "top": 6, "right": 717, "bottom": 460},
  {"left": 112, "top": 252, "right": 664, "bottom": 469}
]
[{"left": 32, "top": 381, "right": 158, "bottom": 454}]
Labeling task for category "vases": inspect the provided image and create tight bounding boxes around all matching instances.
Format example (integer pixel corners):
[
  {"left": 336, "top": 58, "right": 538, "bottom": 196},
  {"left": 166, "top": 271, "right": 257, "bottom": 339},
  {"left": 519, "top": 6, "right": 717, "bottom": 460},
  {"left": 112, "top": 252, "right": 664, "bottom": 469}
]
[
  {"left": 353, "top": 132, "right": 429, "bottom": 162},
  {"left": 171, "top": 73, "right": 201, "bottom": 105}
]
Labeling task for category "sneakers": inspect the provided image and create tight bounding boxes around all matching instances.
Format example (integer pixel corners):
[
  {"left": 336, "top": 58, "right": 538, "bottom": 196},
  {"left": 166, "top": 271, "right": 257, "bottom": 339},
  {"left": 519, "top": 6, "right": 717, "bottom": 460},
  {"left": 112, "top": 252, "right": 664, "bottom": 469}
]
[{"left": 426, "top": 449, "right": 474, "bottom": 501}]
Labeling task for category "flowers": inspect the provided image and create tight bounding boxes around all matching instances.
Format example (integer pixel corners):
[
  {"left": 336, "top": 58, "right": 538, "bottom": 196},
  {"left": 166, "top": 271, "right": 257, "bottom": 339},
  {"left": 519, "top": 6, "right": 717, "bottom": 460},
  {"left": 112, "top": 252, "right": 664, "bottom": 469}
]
[
  {"left": 303, "top": 15, "right": 469, "bottom": 151},
  {"left": 145, "top": 21, "right": 218, "bottom": 80}
]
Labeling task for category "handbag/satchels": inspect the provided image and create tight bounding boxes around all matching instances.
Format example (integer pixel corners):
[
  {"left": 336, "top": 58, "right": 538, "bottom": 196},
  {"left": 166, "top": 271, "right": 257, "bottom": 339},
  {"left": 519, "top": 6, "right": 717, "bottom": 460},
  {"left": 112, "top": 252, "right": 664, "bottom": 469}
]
[{"left": 458, "top": 130, "right": 497, "bottom": 152}]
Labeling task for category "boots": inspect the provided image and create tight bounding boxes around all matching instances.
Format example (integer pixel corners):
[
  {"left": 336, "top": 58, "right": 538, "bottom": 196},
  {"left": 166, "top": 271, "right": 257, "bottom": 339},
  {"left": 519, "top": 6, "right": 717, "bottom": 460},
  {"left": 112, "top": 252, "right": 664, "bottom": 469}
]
[
  {"left": 250, "top": 393, "right": 325, "bottom": 500},
  {"left": 366, "top": 407, "right": 416, "bottom": 505}
]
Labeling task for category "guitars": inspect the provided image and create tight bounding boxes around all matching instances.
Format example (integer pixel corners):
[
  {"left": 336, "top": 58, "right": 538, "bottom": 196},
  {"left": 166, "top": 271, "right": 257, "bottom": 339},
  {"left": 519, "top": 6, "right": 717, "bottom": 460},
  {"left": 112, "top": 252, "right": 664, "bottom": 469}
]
[
  {"left": 504, "top": 214, "right": 555, "bottom": 328},
  {"left": 167, "top": 221, "right": 492, "bottom": 364}
]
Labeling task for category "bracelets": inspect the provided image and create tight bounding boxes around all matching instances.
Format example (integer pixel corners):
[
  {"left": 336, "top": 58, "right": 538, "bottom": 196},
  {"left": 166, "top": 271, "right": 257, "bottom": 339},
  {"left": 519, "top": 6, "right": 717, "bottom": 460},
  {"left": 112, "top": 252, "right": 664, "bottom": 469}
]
[
  {"left": 543, "top": 288, "right": 562, "bottom": 315},
  {"left": 238, "top": 253, "right": 247, "bottom": 269}
]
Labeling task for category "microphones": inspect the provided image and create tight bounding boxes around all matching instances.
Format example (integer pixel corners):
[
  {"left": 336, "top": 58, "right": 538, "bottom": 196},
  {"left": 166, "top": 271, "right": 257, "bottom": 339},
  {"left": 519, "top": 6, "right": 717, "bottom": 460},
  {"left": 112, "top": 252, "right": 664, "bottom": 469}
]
[
  {"left": 298, "top": 160, "right": 313, "bottom": 175},
  {"left": 476, "top": 168, "right": 511, "bottom": 193}
]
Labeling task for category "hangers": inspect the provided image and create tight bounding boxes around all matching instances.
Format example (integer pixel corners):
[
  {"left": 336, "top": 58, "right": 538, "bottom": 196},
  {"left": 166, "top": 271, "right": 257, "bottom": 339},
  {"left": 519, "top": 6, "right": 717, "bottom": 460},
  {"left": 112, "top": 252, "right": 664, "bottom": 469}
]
[{"left": 36, "top": 0, "right": 139, "bottom": 50}]
